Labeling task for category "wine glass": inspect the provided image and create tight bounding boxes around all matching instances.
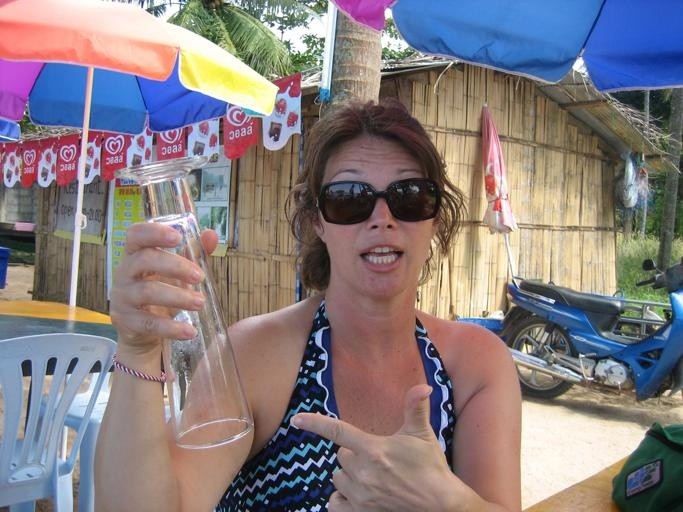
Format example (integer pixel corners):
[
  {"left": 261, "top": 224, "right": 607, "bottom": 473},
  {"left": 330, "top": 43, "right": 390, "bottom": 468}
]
[{"left": 111, "top": 151, "right": 254, "bottom": 453}]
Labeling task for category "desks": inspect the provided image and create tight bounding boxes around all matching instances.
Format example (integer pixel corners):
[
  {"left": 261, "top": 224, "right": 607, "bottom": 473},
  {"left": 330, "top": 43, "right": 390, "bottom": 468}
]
[
  {"left": 0, "top": 296, "right": 118, "bottom": 439},
  {"left": 519, "top": 451, "right": 635, "bottom": 511}
]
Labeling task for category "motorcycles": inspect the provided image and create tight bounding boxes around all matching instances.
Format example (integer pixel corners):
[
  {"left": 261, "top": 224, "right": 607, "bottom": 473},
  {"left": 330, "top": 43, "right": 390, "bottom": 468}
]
[{"left": 494, "top": 255, "right": 683, "bottom": 403}]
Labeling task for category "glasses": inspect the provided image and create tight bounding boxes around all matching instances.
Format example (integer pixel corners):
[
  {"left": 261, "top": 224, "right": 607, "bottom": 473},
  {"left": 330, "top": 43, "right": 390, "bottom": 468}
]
[{"left": 315, "top": 177, "right": 443, "bottom": 223}]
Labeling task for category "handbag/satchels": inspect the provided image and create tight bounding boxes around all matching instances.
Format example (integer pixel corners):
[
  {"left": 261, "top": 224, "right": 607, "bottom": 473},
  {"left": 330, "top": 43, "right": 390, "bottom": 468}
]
[{"left": 611, "top": 422, "right": 682, "bottom": 512}]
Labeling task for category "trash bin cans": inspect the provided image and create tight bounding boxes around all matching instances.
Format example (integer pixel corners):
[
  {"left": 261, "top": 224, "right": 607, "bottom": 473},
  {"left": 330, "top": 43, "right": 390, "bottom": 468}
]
[
  {"left": 457, "top": 318, "right": 524, "bottom": 352},
  {"left": 0, "top": 247, "right": 10, "bottom": 289}
]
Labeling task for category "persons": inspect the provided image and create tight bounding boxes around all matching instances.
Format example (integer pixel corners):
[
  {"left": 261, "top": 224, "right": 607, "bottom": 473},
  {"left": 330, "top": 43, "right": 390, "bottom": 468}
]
[{"left": 93, "top": 97, "right": 521, "bottom": 512}]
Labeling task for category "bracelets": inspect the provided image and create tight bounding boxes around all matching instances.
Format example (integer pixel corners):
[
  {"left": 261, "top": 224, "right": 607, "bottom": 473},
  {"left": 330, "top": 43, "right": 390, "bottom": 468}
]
[{"left": 110, "top": 353, "right": 167, "bottom": 384}]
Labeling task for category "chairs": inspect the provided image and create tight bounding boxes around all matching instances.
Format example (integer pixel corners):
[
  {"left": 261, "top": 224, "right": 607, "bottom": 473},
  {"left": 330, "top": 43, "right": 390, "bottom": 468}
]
[
  {"left": 0, "top": 331, "right": 118, "bottom": 510},
  {"left": 38, "top": 309, "right": 195, "bottom": 512},
  {"left": 33, "top": 369, "right": 181, "bottom": 512}
]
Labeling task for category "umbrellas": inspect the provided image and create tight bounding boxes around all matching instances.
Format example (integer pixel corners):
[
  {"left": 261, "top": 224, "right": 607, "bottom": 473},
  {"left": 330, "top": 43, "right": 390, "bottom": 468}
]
[
  {"left": 481, "top": 103, "right": 516, "bottom": 276},
  {"left": 0, "top": 0, "right": 278, "bottom": 311},
  {"left": 332, "top": 0, "right": 683, "bottom": 93}
]
